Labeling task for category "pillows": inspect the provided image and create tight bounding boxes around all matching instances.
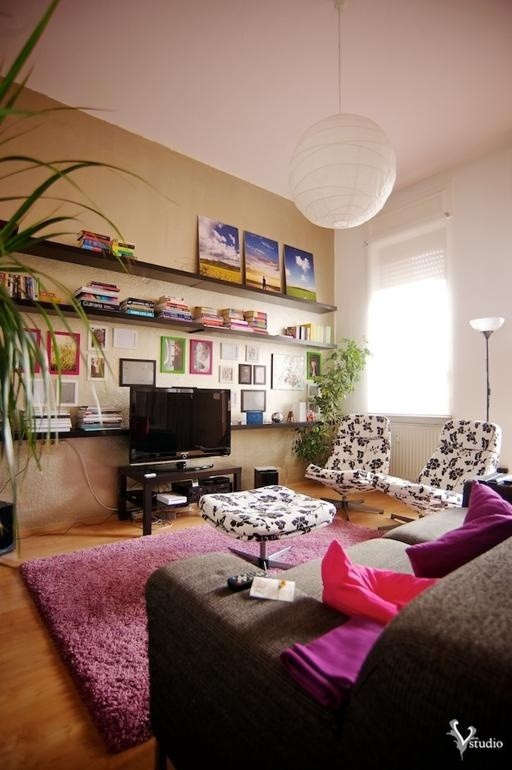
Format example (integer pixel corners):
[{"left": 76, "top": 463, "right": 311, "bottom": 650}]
[
  {"left": 320, "top": 540, "right": 442, "bottom": 627},
  {"left": 404, "top": 479, "right": 512, "bottom": 579}
]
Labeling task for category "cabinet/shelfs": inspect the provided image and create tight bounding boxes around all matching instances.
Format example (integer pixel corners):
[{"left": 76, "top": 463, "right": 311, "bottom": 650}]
[
  {"left": 0, "top": 229, "right": 345, "bottom": 441},
  {"left": 115, "top": 465, "right": 241, "bottom": 536}
]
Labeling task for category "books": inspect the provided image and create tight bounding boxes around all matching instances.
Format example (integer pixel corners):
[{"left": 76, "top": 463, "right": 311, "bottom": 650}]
[
  {"left": 287, "top": 323, "right": 333, "bottom": 346},
  {"left": 1, "top": 271, "right": 267, "bottom": 335},
  {"left": 0, "top": 405, "right": 122, "bottom": 432},
  {"left": 77, "top": 229, "right": 136, "bottom": 261}
]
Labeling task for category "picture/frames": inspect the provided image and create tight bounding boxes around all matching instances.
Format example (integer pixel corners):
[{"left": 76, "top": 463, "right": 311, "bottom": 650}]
[
  {"left": 271, "top": 351, "right": 324, "bottom": 413},
  {"left": 86, "top": 325, "right": 113, "bottom": 382},
  {"left": 10, "top": 327, "right": 41, "bottom": 374},
  {"left": 160, "top": 335, "right": 186, "bottom": 374},
  {"left": 219, "top": 341, "right": 267, "bottom": 413},
  {"left": 113, "top": 327, "right": 138, "bottom": 350},
  {"left": 47, "top": 330, "right": 80, "bottom": 375},
  {"left": 189, "top": 339, "right": 213, "bottom": 374},
  {"left": 119, "top": 357, "right": 157, "bottom": 387}
]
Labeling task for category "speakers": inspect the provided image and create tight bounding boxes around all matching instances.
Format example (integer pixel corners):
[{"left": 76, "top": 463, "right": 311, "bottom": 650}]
[
  {"left": 0, "top": 501, "right": 15, "bottom": 555},
  {"left": 255, "top": 467, "right": 278, "bottom": 488}
]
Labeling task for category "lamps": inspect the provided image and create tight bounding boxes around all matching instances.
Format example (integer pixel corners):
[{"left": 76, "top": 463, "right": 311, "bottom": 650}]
[
  {"left": 287, "top": 0, "right": 398, "bottom": 229},
  {"left": 469, "top": 316, "right": 505, "bottom": 421}
]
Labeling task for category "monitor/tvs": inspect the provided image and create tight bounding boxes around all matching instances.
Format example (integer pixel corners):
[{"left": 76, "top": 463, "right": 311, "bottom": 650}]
[{"left": 130, "top": 384, "right": 231, "bottom": 472}]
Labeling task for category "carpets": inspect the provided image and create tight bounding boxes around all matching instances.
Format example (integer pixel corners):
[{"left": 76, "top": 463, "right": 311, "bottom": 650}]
[{"left": 17, "top": 516, "right": 385, "bottom": 754}]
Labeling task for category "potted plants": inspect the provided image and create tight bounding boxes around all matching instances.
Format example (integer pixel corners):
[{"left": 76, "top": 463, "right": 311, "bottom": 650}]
[{"left": 1, "top": 2, "right": 179, "bottom": 555}]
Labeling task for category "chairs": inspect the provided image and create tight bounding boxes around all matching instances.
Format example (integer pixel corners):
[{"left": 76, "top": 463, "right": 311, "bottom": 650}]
[
  {"left": 304, "top": 413, "right": 392, "bottom": 521},
  {"left": 373, "top": 417, "right": 502, "bottom": 532}
]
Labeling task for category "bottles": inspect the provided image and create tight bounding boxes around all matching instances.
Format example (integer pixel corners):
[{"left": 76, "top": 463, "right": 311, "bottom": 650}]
[
  {"left": 306, "top": 410, "right": 314, "bottom": 424},
  {"left": 287, "top": 410, "right": 295, "bottom": 424}
]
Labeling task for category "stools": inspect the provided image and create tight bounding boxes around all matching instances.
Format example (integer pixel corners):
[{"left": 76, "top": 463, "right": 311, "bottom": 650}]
[{"left": 197, "top": 484, "right": 337, "bottom": 575}]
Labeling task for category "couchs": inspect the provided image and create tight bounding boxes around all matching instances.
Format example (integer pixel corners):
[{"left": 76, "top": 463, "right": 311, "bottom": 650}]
[{"left": 144, "top": 500, "right": 512, "bottom": 768}]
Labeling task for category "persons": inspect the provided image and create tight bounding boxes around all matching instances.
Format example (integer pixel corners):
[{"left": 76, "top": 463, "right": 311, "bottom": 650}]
[
  {"left": 92, "top": 329, "right": 104, "bottom": 348},
  {"left": 91, "top": 359, "right": 104, "bottom": 377},
  {"left": 310, "top": 356, "right": 318, "bottom": 377}
]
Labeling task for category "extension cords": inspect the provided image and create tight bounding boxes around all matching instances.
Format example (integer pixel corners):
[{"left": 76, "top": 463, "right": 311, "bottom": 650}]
[{"left": 131, "top": 512, "right": 143, "bottom": 519}]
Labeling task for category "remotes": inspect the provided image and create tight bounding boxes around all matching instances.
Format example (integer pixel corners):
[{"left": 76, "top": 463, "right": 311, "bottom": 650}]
[{"left": 228, "top": 571, "right": 266, "bottom": 589}]
